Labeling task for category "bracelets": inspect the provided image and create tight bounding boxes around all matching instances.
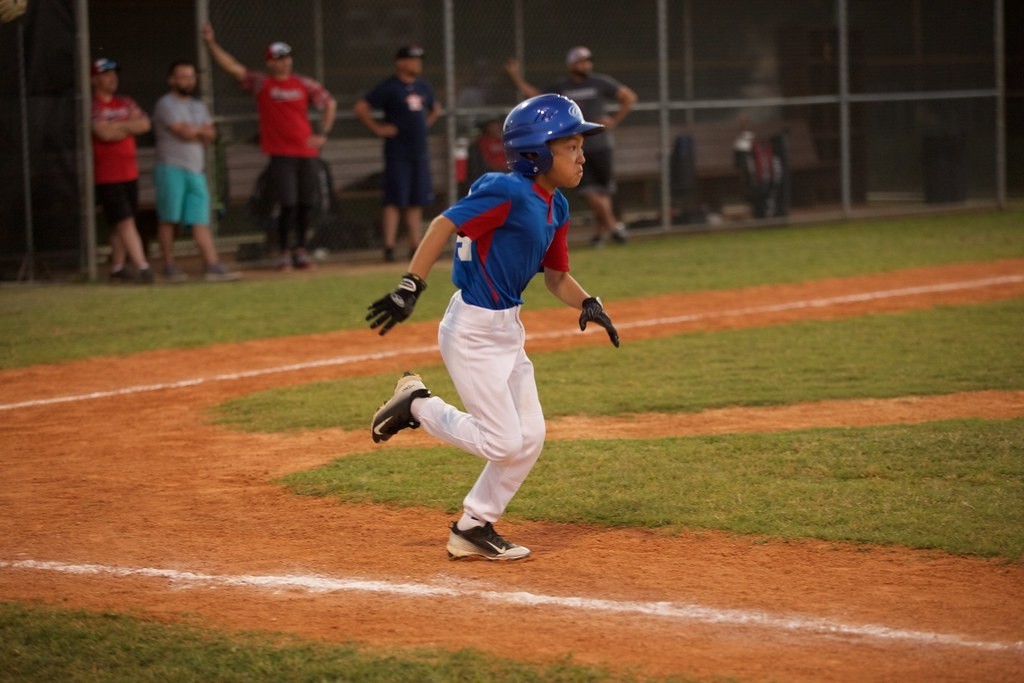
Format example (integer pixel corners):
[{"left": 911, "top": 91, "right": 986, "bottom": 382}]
[{"left": 318, "top": 129, "right": 330, "bottom": 137}]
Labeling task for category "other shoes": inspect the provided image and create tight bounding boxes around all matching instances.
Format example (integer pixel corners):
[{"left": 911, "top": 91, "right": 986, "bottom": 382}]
[
  {"left": 383, "top": 246, "right": 396, "bottom": 263},
  {"left": 203, "top": 263, "right": 242, "bottom": 282},
  {"left": 132, "top": 266, "right": 153, "bottom": 285},
  {"left": 589, "top": 234, "right": 602, "bottom": 249},
  {"left": 162, "top": 263, "right": 195, "bottom": 284},
  {"left": 609, "top": 221, "right": 628, "bottom": 245},
  {"left": 275, "top": 246, "right": 315, "bottom": 274},
  {"left": 109, "top": 267, "right": 133, "bottom": 280},
  {"left": 409, "top": 248, "right": 418, "bottom": 261}
]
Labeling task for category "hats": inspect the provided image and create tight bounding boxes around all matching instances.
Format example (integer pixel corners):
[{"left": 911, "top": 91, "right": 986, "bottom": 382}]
[
  {"left": 396, "top": 46, "right": 425, "bottom": 59},
  {"left": 91, "top": 58, "right": 117, "bottom": 76},
  {"left": 565, "top": 46, "right": 593, "bottom": 65},
  {"left": 265, "top": 42, "right": 294, "bottom": 62}
]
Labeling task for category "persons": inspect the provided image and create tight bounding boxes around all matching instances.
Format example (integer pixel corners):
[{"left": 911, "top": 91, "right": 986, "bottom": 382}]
[
  {"left": 365, "top": 94, "right": 619, "bottom": 561},
  {"left": 203, "top": 22, "right": 337, "bottom": 275},
  {"left": 353, "top": 43, "right": 443, "bottom": 264},
  {"left": 150, "top": 60, "right": 243, "bottom": 283},
  {"left": 90, "top": 58, "right": 169, "bottom": 285},
  {"left": 506, "top": 46, "right": 636, "bottom": 250}
]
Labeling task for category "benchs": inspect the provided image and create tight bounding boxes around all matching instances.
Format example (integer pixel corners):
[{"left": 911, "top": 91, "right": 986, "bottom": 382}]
[{"left": 78, "top": 119, "right": 823, "bottom": 264}]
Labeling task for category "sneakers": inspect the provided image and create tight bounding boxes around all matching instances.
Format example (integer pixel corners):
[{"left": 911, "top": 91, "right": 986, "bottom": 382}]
[
  {"left": 370, "top": 371, "right": 431, "bottom": 444},
  {"left": 445, "top": 520, "right": 531, "bottom": 563}
]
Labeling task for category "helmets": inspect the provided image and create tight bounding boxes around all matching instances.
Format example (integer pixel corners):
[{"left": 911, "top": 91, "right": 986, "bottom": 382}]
[{"left": 502, "top": 93, "right": 606, "bottom": 178}]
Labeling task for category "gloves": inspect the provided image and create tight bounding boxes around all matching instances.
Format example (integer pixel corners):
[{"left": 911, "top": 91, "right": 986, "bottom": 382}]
[
  {"left": 365, "top": 273, "right": 428, "bottom": 336},
  {"left": 579, "top": 296, "right": 619, "bottom": 348}
]
